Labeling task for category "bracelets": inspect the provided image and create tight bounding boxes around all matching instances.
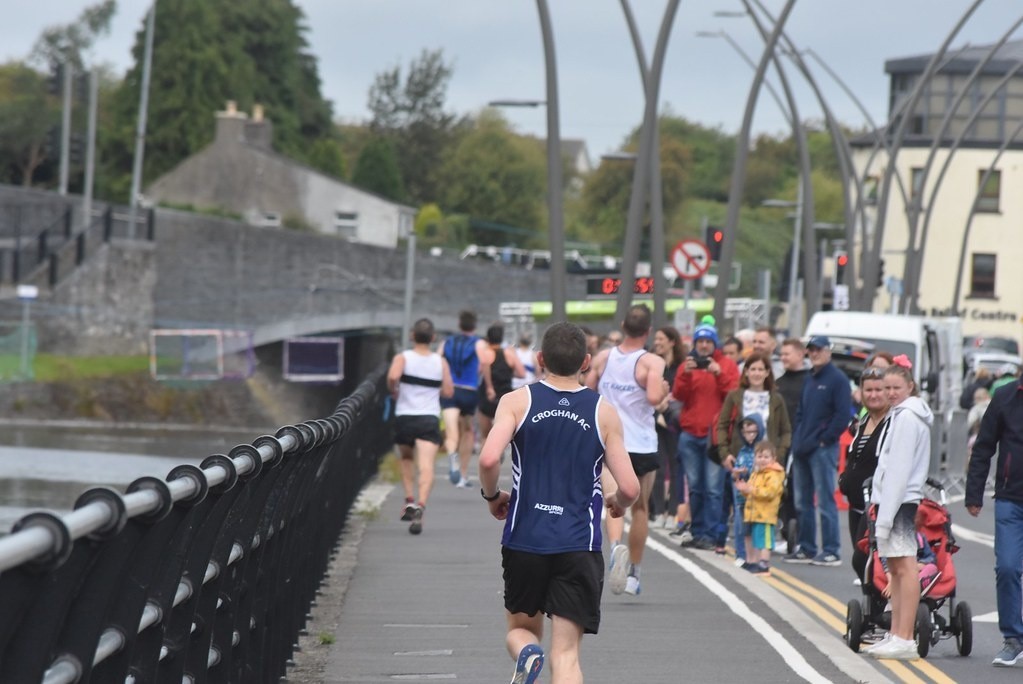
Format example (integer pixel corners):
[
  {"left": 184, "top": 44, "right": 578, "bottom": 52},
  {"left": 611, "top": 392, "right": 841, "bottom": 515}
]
[{"left": 481, "top": 485, "right": 500, "bottom": 501}]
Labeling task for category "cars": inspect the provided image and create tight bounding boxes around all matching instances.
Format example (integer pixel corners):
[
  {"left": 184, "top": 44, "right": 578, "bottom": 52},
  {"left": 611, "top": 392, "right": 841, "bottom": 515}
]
[{"left": 961, "top": 337, "right": 1022, "bottom": 418}]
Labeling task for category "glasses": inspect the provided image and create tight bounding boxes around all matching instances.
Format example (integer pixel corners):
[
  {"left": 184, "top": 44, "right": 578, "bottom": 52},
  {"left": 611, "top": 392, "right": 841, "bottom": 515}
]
[{"left": 862, "top": 368, "right": 883, "bottom": 378}]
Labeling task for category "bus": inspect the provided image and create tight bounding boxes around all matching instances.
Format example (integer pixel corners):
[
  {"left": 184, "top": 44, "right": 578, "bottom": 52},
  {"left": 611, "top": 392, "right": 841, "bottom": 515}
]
[{"left": 516, "top": 300, "right": 739, "bottom": 352}]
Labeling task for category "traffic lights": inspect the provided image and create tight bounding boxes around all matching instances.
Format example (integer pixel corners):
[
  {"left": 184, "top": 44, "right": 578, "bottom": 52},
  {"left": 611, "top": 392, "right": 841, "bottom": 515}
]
[
  {"left": 834, "top": 254, "right": 847, "bottom": 282},
  {"left": 702, "top": 224, "right": 725, "bottom": 262}
]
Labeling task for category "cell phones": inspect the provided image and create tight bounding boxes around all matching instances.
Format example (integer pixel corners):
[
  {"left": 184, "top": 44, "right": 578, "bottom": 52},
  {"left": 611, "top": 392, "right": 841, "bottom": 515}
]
[{"left": 695, "top": 360, "right": 710, "bottom": 369}]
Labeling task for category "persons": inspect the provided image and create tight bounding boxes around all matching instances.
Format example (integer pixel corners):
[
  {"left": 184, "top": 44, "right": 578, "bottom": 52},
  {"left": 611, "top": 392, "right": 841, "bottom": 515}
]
[
  {"left": 836, "top": 370, "right": 863, "bottom": 513},
  {"left": 864, "top": 365, "right": 932, "bottom": 662},
  {"left": 963, "top": 371, "right": 1023, "bottom": 667},
  {"left": 779, "top": 334, "right": 852, "bottom": 566},
  {"left": 579, "top": 323, "right": 814, "bottom": 578},
  {"left": 478, "top": 320, "right": 641, "bottom": 684},
  {"left": 837, "top": 351, "right": 899, "bottom": 645},
  {"left": 385, "top": 317, "right": 455, "bottom": 534},
  {"left": 959, "top": 362, "right": 1023, "bottom": 488},
  {"left": 436, "top": 312, "right": 496, "bottom": 492},
  {"left": 475, "top": 322, "right": 543, "bottom": 466},
  {"left": 584, "top": 305, "right": 670, "bottom": 595}
]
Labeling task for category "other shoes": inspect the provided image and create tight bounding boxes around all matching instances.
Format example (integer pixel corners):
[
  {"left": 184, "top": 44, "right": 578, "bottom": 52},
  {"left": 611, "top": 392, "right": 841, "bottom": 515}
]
[
  {"left": 749, "top": 560, "right": 770, "bottom": 575},
  {"left": 510, "top": 644, "right": 544, "bottom": 684},
  {"left": 400, "top": 498, "right": 424, "bottom": 536},
  {"left": 734, "top": 557, "right": 745, "bottom": 567},
  {"left": 742, "top": 562, "right": 752, "bottom": 570},
  {"left": 450, "top": 454, "right": 472, "bottom": 490},
  {"left": 653, "top": 514, "right": 729, "bottom": 555}
]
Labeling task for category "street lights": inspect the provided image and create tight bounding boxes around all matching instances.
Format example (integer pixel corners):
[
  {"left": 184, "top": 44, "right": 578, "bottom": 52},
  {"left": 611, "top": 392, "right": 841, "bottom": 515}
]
[
  {"left": 37, "top": 24, "right": 99, "bottom": 224},
  {"left": 703, "top": 4, "right": 920, "bottom": 327}
]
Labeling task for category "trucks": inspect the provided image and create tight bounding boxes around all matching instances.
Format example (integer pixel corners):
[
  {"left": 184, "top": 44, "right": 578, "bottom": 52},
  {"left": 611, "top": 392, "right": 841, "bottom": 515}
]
[{"left": 800, "top": 310, "right": 960, "bottom": 436}]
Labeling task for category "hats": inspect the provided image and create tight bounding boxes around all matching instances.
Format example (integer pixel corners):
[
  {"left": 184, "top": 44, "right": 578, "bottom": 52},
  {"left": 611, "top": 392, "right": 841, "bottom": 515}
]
[
  {"left": 694, "top": 322, "right": 719, "bottom": 349},
  {"left": 805, "top": 335, "right": 830, "bottom": 349}
]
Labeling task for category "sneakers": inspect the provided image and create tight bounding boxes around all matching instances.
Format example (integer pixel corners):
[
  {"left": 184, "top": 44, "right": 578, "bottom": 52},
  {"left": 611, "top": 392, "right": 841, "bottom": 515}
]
[
  {"left": 860, "top": 633, "right": 920, "bottom": 660},
  {"left": 607, "top": 543, "right": 642, "bottom": 595},
  {"left": 920, "top": 570, "right": 941, "bottom": 598},
  {"left": 992, "top": 638, "right": 1023, "bottom": 666},
  {"left": 810, "top": 554, "right": 843, "bottom": 566},
  {"left": 783, "top": 549, "right": 817, "bottom": 563}
]
